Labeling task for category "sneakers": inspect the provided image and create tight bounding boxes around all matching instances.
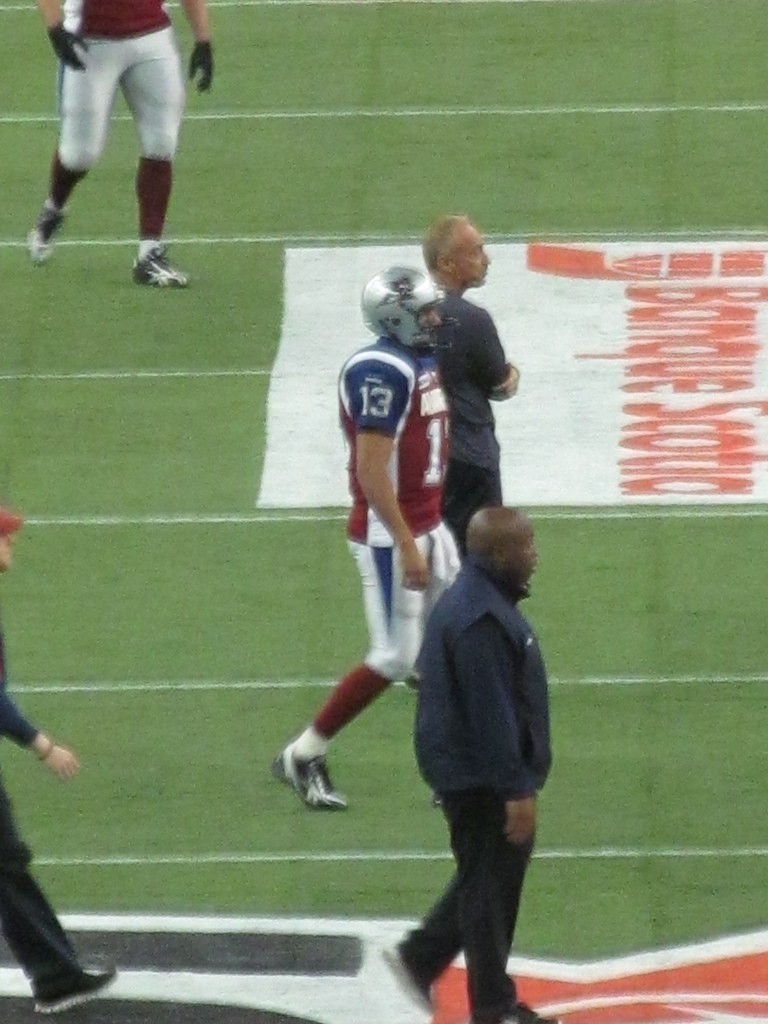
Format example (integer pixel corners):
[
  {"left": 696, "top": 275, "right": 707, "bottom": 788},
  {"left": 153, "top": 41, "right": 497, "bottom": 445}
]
[
  {"left": 132, "top": 241, "right": 189, "bottom": 288},
  {"left": 273, "top": 742, "right": 350, "bottom": 812},
  {"left": 28, "top": 199, "right": 69, "bottom": 266}
]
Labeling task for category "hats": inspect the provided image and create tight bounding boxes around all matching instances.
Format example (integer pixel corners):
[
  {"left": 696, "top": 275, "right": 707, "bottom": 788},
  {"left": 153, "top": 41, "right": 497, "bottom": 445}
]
[{"left": 0, "top": 508, "right": 23, "bottom": 536}]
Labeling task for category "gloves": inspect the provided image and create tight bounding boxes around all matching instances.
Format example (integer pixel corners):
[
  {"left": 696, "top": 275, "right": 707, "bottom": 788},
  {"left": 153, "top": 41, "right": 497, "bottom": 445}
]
[
  {"left": 188, "top": 40, "right": 214, "bottom": 91},
  {"left": 47, "top": 22, "right": 89, "bottom": 72}
]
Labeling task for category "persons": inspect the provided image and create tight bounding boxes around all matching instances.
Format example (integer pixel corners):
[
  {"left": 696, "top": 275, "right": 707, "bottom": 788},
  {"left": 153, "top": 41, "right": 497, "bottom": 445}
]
[
  {"left": 29, "top": 0, "right": 214, "bottom": 289},
  {"left": 0, "top": 510, "right": 119, "bottom": 1013},
  {"left": 423, "top": 216, "right": 521, "bottom": 548},
  {"left": 271, "top": 264, "right": 460, "bottom": 810},
  {"left": 384, "top": 509, "right": 564, "bottom": 1024}
]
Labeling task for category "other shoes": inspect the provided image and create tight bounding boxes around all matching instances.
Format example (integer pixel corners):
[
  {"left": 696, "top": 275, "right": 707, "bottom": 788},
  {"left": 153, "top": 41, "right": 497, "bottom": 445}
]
[
  {"left": 34, "top": 954, "right": 118, "bottom": 1014},
  {"left": 469, "top": 1003, "right": 562, "bottom": 1024},
  {"left": 383, "top": 945, "right": 436, "bottom": 1017}
]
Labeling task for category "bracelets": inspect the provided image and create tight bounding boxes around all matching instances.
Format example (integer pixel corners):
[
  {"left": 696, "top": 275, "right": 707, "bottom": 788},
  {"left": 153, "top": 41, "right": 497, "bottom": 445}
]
[{"left": 39, "top": 743, "right": 53, "bottom": 760}]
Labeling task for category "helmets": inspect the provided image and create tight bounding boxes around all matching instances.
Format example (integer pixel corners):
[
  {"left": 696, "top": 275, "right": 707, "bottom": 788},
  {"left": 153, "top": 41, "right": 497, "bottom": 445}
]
[{"left": 361, "top": 267, "right": 461, "bottom": 350}]
